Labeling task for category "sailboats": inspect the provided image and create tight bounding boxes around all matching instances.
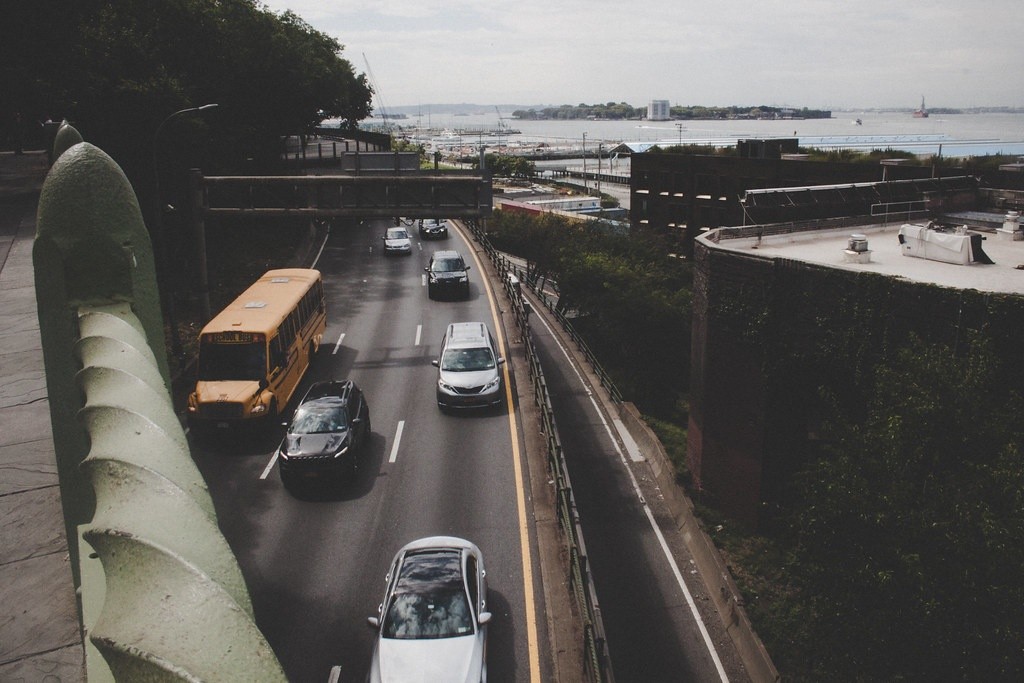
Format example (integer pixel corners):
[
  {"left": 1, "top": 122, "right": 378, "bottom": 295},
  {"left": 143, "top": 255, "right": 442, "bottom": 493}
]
[{"left": 390, "top": 106, "right": 592, "bottom": 160}]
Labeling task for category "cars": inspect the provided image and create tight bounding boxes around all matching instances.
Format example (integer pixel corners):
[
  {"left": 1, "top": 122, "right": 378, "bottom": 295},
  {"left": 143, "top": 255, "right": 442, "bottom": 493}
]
[
  {"left": 382, "top": 227, "right": 414, "bottom": 255},
  {"left": 368, "top": 534, "right": 493, "bottom": 683},
  {"left": 417, "top": 218, "right": 448, "bottom": 239}
]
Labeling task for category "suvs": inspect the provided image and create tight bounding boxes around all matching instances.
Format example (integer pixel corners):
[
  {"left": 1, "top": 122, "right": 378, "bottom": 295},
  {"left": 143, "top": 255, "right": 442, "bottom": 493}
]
[
  {"left": 425, "top": 251, "right": 471, "bottom": 299},
  {"left": 278, "top": 378, "right": 371, "bottom": 494},
  {"left": 434, "top": 321, "right": 506, "bottom": 416}
]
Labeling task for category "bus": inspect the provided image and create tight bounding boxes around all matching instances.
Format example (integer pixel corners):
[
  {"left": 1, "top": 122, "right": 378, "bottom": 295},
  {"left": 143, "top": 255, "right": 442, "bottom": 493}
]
[{"left": 185, "top": 267, "right": 336, "bottom": 429}]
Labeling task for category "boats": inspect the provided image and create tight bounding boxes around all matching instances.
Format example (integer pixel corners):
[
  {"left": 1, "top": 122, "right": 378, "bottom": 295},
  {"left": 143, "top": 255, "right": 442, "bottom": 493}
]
[{"left": 912, "top": 98, "right": 929, "bottom": 119}]
